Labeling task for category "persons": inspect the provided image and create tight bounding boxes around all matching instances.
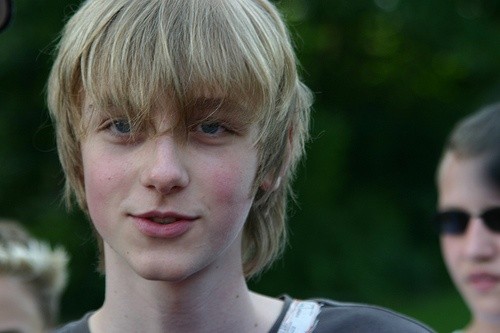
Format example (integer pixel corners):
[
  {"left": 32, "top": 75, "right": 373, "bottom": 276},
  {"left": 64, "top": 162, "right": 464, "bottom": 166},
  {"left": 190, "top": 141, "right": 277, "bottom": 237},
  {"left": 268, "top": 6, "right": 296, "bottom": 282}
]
[
  {"left": 437, "top": 103, "right": 500, "bottom": 331},
  {"left": 1, "top": 221, "right": 69, "bottom": 333},
  {"left": 46, "top": 0, "right": 439, "bottom": 332}
]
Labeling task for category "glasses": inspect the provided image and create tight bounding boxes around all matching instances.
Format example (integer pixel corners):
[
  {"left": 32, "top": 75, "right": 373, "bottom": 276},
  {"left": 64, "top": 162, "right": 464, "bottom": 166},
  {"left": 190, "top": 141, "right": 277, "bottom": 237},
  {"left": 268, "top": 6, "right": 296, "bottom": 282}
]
[{"left": 433, "top": 206, "right": 500, "bottom": 236}]
